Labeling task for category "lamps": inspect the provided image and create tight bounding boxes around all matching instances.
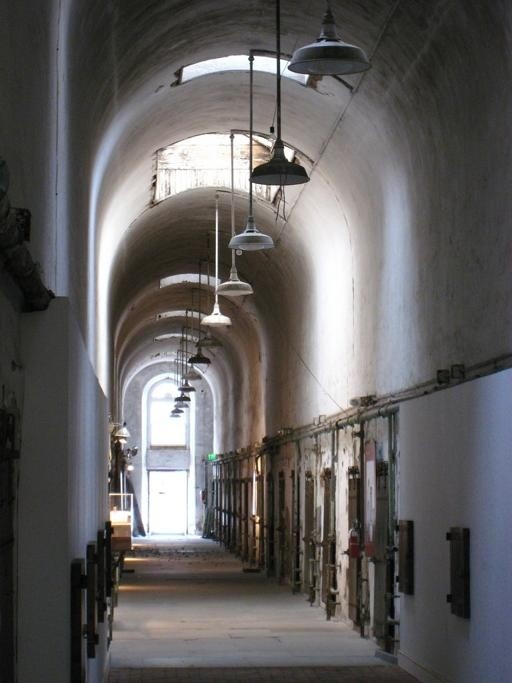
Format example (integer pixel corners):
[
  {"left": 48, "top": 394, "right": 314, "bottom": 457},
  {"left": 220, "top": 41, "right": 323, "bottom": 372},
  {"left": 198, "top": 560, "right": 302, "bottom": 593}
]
[
  {"left": 198, "top": 133, "right": 254, "bottom": 328},
  {"left": 7, "top": 275, "right": 53, "bottom": 313},
  {"left": 168, "top": 309, "right": 202, "bottom": 418},
  {"left": 229, "top": 1, "right": 309, "bottom": 250},
  {"left": 288, "top": 2, "right": 370, "bottom": 76},
  {"left": 188, "top": 234, "right": 221, "bottom": 365}
]
